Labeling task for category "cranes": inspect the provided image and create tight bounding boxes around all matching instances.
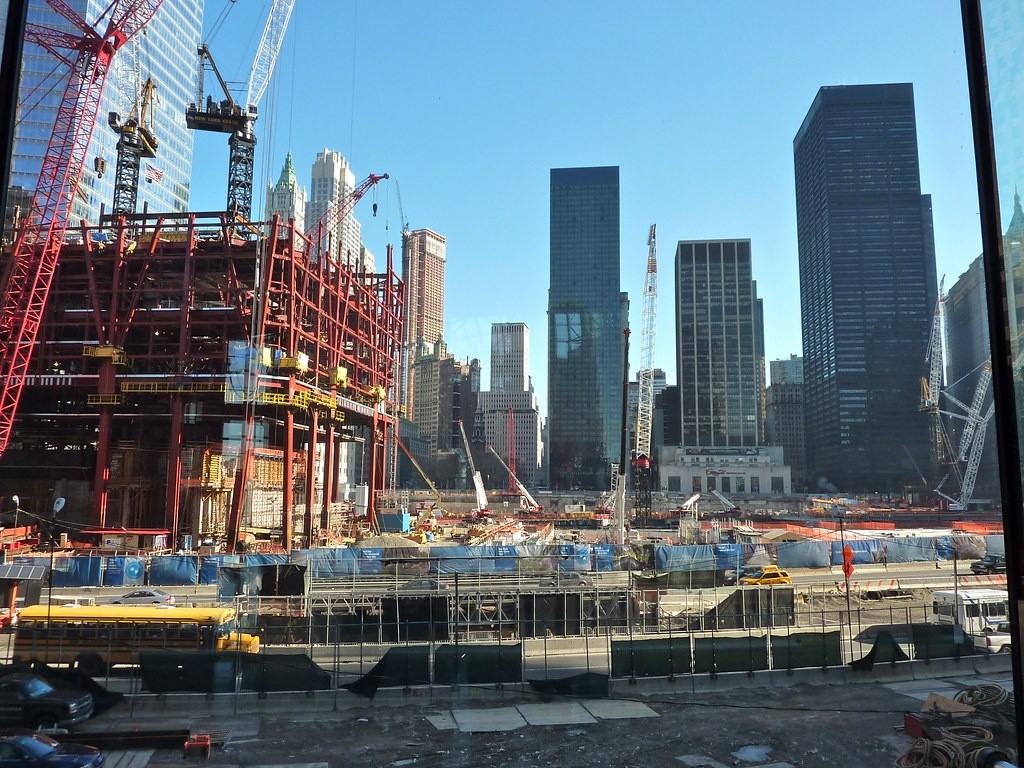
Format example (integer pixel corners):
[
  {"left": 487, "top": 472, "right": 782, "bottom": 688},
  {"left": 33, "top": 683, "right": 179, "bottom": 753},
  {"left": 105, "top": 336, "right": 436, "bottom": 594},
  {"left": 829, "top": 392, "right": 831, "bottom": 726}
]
[{"left": 0, "top": 1, "right": 1021, "bottom": 556}]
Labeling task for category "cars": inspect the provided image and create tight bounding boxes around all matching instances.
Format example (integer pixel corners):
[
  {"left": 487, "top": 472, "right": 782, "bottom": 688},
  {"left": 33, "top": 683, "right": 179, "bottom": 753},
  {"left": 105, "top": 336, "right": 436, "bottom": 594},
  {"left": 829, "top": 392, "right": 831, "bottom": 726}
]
[
  {"left": 736, "top": 565, "right": 792, "bottom": 585},
  {"left": 386, "top": 579, "right": 449, "bottom": 591},
  {"left": 108, "top": 590, "right": 176, "bottom": 606},
  {"left": 540, "top": 571, "right": 594, "bottom": 586},
  {"left": 0, "top": 732, "right": 104, "bottom": 768},
  {"left": 0, "top": 672, "right": 95, "bottom": 728},
  {"left": 970, "top": 553, "right": 1006, "bottom": 575}
]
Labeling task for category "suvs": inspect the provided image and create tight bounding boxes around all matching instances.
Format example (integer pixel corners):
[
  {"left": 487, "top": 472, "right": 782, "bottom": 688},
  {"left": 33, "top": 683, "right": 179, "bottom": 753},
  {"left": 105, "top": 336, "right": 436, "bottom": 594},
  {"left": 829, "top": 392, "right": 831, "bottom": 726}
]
[{"left": 721, "top": 566, "right": 763, "bottom": 586}]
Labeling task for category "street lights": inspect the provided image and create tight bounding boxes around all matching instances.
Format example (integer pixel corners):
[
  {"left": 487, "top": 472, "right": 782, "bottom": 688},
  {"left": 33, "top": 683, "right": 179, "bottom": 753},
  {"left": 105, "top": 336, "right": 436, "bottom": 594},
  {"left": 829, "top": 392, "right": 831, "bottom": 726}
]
[
  {"left": 43, "top": 498, "right": 66, "bottom": 671},
  {"left": 9, "top": 492, "right": 19, "bottom": 563}
]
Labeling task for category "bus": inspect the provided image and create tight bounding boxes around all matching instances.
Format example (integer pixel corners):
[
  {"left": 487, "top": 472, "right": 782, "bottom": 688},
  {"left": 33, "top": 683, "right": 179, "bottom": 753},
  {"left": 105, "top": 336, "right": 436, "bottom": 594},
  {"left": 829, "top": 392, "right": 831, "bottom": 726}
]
[{"left": 12, "top": 605, "right": 260, "bottom": 677}]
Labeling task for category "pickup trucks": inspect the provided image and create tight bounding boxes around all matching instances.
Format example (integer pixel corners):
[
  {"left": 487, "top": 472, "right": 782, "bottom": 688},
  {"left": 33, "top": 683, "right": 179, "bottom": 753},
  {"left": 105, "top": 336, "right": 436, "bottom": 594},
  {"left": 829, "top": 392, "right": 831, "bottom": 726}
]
[{"left": 973, "top": 621, "right": 1012, "bottom": 653}]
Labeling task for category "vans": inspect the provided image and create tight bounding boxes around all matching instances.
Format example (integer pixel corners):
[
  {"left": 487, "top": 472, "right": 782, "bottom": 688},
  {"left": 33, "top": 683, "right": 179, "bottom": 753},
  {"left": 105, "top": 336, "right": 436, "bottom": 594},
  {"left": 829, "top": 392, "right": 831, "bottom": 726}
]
[{"left": 933, "top": 589, "right": 1009, "bottom": 633}]
